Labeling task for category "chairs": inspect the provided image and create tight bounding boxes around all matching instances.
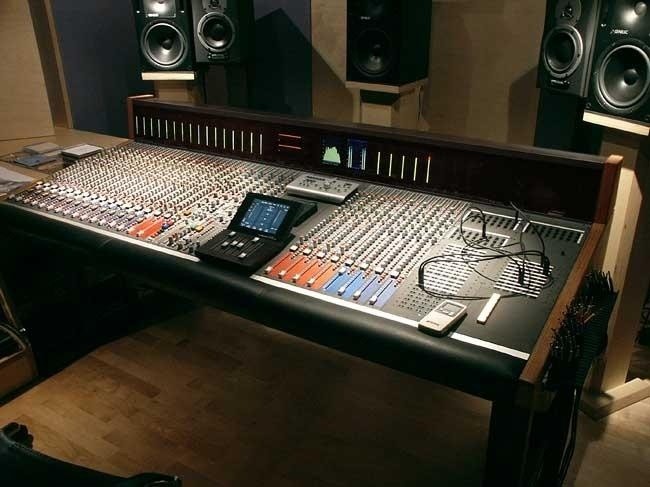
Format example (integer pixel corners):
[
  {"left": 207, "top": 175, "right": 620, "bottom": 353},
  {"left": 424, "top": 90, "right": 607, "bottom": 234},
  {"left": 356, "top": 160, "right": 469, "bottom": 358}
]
[{"left": 0, "top": 421, "right": 183, "bottom": 486}]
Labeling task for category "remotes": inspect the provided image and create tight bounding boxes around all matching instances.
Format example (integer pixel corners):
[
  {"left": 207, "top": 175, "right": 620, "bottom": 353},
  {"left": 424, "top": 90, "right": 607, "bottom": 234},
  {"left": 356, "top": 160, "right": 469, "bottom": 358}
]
[{"left": 418, "top": 299, "right": 468, "bottom": 336}]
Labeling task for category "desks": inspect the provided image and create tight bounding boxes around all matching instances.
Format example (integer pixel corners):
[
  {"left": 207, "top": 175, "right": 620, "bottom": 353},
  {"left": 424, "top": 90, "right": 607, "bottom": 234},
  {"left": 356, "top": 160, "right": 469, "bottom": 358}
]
[
  {"left": 140, "top": 70, "right": 207, "bottom": 104},
  {"left": 578, "top": 108, "right": 650, "bottom": 423},
  {"left": 344, "top": 76, "right": 429, "bottom": 131}
]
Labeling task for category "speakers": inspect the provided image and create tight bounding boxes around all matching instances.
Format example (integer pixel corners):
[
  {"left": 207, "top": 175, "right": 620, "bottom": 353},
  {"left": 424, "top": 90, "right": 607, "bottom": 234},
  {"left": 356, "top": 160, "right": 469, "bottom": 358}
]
[
  {"left": 586, "top": 0, "right": 650, "bottom": 126},
  {"left": 131, "top": 0, "right": 196, "bottom": 71},
  {"left": 190, "top": 0, "right": 253, "bottom": 64},
  {"left": 346, "top": 0, "right": 431, "bottom": 87},
  {"left": 534, "top": 0, "right": 598, "bottom": 99}
]
